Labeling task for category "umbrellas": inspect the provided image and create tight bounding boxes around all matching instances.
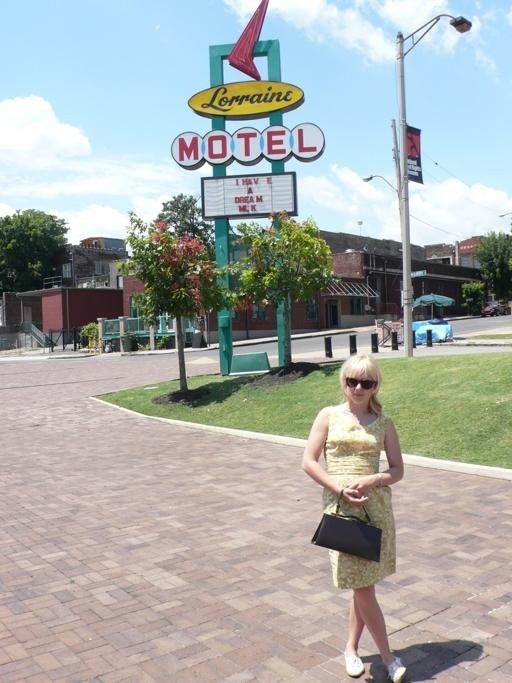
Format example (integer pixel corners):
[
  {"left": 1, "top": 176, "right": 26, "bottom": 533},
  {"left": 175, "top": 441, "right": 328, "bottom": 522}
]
[{"left": 411, "top": 292, "right": 456, "bottom": 321}]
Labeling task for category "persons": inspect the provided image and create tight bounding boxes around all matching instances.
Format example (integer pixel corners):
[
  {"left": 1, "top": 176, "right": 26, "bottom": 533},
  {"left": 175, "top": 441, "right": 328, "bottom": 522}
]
[{"left": 299, "top": 352, "right": 409, "bottom": 683}]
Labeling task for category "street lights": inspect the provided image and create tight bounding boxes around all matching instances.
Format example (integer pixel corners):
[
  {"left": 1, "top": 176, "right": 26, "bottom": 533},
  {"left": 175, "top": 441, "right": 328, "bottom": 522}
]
[{"left": 362, "top": 12, "right": 473, "bottom": 358}]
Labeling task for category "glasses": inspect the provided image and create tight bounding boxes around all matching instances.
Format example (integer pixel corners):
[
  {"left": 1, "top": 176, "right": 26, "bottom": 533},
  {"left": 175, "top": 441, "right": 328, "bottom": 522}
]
[{"left": 345, "top": 377, "right": 375, "bottom": 389}]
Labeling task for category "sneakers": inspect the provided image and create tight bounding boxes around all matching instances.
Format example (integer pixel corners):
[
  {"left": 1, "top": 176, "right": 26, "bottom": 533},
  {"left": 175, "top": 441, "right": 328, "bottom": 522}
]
[
  {"left": 344, "top": 650, "right": 365, "bottom": 676},
  {"left": 388, "top": 655, "right": 407, "bottom": 683}
]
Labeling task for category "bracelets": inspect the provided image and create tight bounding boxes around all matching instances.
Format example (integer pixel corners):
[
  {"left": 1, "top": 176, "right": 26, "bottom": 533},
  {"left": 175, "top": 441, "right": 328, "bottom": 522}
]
[{"left": 375, "top": 471, "right": 386, "bottom": 489}]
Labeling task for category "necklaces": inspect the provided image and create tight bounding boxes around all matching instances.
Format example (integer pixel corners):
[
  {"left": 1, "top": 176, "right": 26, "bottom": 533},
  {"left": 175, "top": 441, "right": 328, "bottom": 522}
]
[{"left": 340, "top": 404, "right": 373, "bottom": 424}]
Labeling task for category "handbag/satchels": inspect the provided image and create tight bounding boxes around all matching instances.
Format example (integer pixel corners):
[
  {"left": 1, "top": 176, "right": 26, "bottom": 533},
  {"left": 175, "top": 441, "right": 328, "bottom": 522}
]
[{"left": 311, "top": 513, "right": 382, "bottom": 562}]
